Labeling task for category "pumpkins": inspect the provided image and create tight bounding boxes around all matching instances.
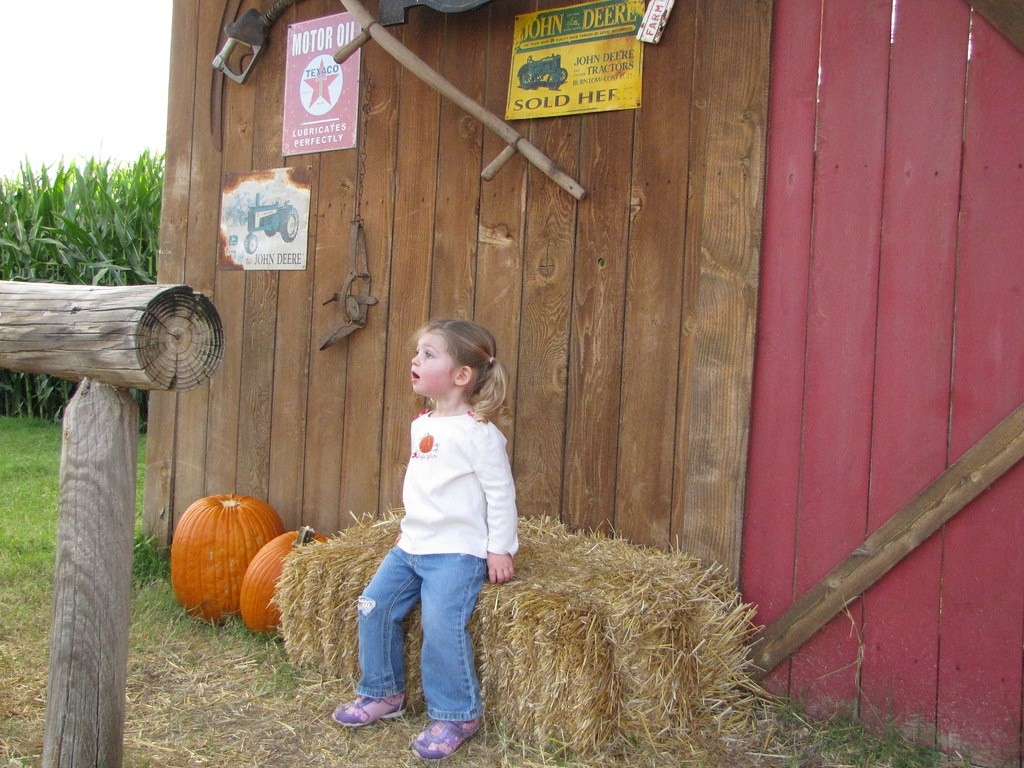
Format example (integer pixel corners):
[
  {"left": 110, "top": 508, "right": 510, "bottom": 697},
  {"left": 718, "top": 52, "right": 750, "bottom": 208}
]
[
  {"left": 170, "top": 493, "right": 286, "bottom": 625},
  {"left": 241, "top": 526, "right": 334, "bottom": 636}
]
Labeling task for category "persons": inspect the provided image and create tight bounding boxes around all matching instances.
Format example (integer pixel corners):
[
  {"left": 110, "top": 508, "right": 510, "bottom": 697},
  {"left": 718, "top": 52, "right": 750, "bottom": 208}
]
[{"left": 332, "top": 319, "right": 519, "bottom": 761}]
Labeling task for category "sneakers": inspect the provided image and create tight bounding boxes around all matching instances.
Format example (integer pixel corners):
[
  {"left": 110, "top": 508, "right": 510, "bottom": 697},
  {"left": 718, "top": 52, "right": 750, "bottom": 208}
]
[
  {"left": 411, "top": 715, "right": 484, "bottom": 762},
  {"left": 332, "top": 690, "right": 408, "bottom": 727}
]
